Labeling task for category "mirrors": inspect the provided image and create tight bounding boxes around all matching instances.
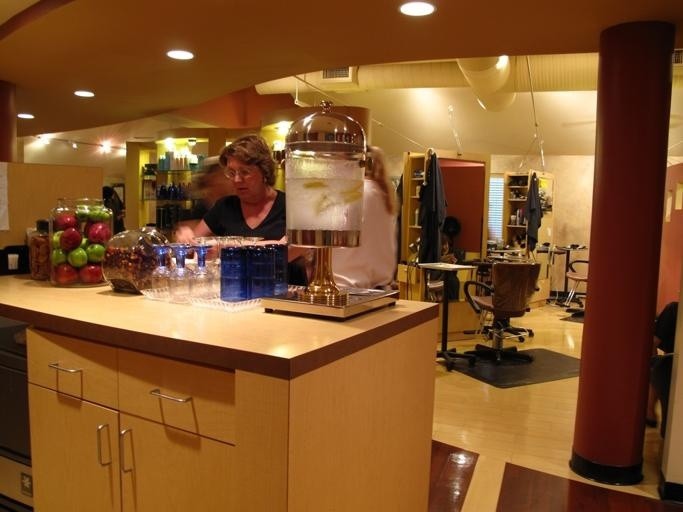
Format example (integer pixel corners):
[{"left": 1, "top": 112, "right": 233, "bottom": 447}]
[
  {"left": 425, "top": 148, "right": 491, "bottom": 302},
  {"left": 533, "top": 170, "right": 553, "bottom": 280}
]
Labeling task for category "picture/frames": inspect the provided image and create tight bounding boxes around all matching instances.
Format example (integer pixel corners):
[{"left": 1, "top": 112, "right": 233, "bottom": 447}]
[{"left": 112, "top": 183, "right": 125, "bottom": 206}]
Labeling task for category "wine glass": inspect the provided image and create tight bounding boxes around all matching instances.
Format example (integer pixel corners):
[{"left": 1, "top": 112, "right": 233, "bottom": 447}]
[{"left": 152, "top": 242, "right": 215, "bottom": 300}]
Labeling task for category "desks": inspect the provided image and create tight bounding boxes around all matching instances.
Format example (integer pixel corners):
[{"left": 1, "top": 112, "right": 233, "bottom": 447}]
[
  {"left": 487, "top": 249, "right": 522, "bottom": 262},
  {"left": 545, "top": 247, "right": 588, "bottom": 306},
  {"left": 415, "top": 263, "right": 476, "bottom": 371}
]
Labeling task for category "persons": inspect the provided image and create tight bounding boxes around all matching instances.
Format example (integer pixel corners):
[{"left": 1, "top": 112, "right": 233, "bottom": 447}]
[
  {"left": 643, "top": 301, "right": 677, "bottom": 437},
  {"left": 102, "top": 186, "right": 126, "bottom": 234},
  {"left": 173, "top": 133, "right": 286, "bottom": 244},
  {"left": 326, "top": 144, "right": 401, "bottom": 292}
]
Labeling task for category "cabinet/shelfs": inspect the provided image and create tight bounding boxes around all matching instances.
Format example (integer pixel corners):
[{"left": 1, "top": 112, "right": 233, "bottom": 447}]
[
  {"left": 21, "top": 303, "right": 439, "bottom": 512},
  {"left": 397, "top": 150, "right": 427, "bottom": 302},
  {"left": 501, "top": 169, "right": 530, "bottom": 251},
  {"left": 153, "top": 128, "right": 209, "bottom": 237}
]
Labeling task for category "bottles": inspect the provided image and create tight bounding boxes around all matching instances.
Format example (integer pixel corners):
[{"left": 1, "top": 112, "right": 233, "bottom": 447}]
[
  {"left": 221, "top": 236, "right": 288, "bottom": 303},
  {"left": 160, "top": 151, "right": 188, "bottom": 169},
  {"left": 29, "top": 198, "right": 170, "bottom": 292},
  {"left": 158, "top": 180, "right": 193, "bottom": 200}
]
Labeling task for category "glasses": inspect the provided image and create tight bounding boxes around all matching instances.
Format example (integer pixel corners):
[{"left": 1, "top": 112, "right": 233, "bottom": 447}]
[{"left": 224, "top": 166, "right": 260, "bottom": 180}]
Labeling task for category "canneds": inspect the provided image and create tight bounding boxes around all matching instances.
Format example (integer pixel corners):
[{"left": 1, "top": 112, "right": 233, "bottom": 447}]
[{"left": 30, "top": 235, "right": 50, "bottom": 281}]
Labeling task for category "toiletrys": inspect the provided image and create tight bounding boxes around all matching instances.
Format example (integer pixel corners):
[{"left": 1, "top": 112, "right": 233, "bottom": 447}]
[{"left": 155, "top": 150, "right": 189, "bottom": 171}]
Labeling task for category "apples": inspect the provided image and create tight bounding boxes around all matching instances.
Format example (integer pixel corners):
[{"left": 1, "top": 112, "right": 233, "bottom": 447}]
[{"left": 50, "top": 204, "right": 112, "bottom": 284}]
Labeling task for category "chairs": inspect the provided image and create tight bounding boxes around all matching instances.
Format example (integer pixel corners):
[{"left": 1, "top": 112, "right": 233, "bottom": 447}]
[
  {"left": 560, "top": 259, "right": 589, "bottom": 318},
  {"left": 462, "top": 262, "right": 542, "bottom": 372}
]
